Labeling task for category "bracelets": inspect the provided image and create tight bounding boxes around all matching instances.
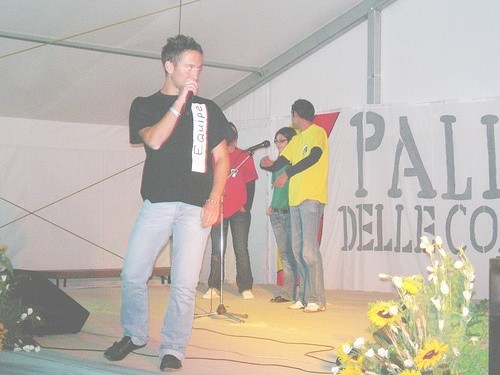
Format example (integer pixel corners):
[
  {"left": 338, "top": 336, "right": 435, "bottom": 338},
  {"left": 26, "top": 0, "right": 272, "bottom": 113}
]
[
  {"left": 169, "top": 106, "right": 182, "bottom": 118},
  {"left": 205, "top": 199, "right": 221, "bottom": 206}
]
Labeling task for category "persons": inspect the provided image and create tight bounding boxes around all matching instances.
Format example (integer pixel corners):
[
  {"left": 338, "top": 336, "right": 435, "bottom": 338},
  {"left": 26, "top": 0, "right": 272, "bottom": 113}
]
[
  {"left": 266, "top": 127, "right": 301, "bottom": 303},
  {"left": 259, "top": 98, "right": 328, "bottom": 313},
  {"left": 105, "top": 34, "right": 237, "bottom": 370},
  {"left": 203, "top": 122, "right": 259, "bottom": 300}
]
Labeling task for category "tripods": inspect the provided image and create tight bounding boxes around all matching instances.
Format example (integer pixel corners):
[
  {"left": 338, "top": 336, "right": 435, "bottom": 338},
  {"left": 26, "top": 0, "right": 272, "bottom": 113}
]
[{"left": 193, "top": 150, "right": 254, "bottom": 324}]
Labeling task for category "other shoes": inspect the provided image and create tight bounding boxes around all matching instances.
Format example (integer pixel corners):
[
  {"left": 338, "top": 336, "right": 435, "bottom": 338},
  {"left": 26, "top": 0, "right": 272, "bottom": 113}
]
[
  {"left": 160, "top": 355, "right": 182, "bottom": 371},
  {"left": 288, "top": 301, "right": 305, "bottom": 310},
  {"left": 304, "top": 303, "right": 325, "bottom": 312},
  {"left": 271, "top": 295, "right": 294, "bottom": 302},
  {"left": 201, "top": 288, "right": 220, "bottom": 298},
  {"left": 241, "top": 290, "right": 254, "bottom": 299},
  {"left": 103, "top": 336, "right": 146, "bottom": 361}
]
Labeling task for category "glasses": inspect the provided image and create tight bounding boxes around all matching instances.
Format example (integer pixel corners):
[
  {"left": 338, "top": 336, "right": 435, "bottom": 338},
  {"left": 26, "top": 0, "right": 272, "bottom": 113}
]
[{"left": 274, "top": 139, "right": 286, "bottom": 144}]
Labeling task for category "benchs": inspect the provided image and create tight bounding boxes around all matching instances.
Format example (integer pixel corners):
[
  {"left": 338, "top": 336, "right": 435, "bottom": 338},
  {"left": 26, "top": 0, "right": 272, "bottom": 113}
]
[{"left": 36, "top": 267, "right": 171, "bottom": 288}]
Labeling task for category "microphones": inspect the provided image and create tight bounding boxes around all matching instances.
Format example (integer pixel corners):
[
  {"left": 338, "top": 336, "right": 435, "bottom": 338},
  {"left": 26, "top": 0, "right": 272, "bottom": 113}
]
[
  {"left": 185, "top": 91, "right": 194, "bottom": 115},
  {"left": 242, "top": 140, "right": 270, "bottom": 153}
]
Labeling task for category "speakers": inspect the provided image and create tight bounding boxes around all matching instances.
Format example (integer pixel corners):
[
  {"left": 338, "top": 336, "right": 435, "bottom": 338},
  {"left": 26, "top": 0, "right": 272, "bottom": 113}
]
[{"left": 10, "top": 269, "right": 90, "bottom": 337}]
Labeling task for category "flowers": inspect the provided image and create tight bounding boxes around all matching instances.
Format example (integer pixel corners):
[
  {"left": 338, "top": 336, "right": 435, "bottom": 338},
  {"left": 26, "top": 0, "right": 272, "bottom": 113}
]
[
  {"left": 0, "top": 244, "right": 43, "bottom": 352},
  {"left": 330, "top": 235, "right": 491, "bottom": 375}
]
